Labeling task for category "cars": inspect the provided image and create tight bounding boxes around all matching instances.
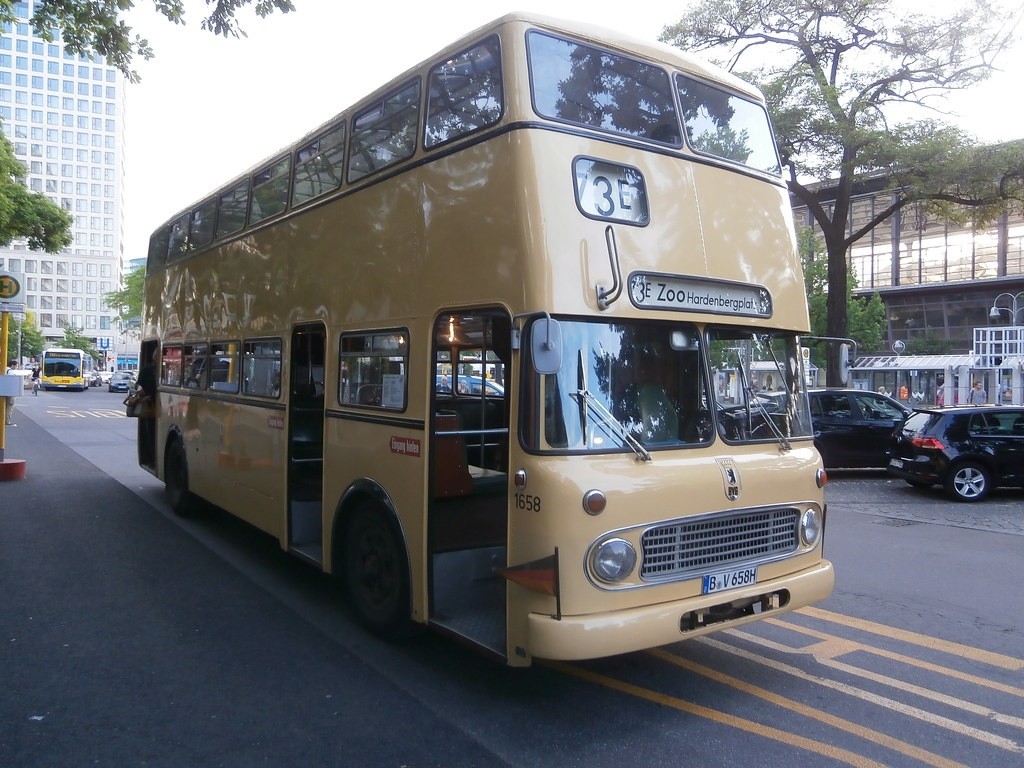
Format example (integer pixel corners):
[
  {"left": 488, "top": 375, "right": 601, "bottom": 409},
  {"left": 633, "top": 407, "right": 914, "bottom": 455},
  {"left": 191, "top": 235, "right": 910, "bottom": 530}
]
[
  {"left": 107, "top": 369, "right": 138, "bottom": 393},
  {"left": 435, "top": 375, "right": 504, "bottom": 397},
  {"left": 90, "top": 371, "right": 102, "bottom": 387}
]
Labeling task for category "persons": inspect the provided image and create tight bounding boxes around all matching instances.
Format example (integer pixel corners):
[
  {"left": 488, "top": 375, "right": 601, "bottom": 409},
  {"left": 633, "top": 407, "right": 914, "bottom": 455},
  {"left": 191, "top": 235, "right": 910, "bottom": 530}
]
[
  {"left": 191, "top": 346, "right": 230, "bottom": 390},
  {"left": 6, "top": 361, "right": 18, "bottom": 426},
  {"left": 437, "top": 375, "right": 450, "bottom": 392},
  {"left": 878, "top": 386, "right": 885, "bottom": 395},
  {"left": 459, "top": 380, "right": 470, "bottom": 394},
  {"left": 968, "top": 380, "right": 986, "bottom": 405},
  {"left": 32, "top": 365, "right": 42, "bottom": 394},
  {"left": 135, "top": 348, "right": 157, "bottom": 461}
]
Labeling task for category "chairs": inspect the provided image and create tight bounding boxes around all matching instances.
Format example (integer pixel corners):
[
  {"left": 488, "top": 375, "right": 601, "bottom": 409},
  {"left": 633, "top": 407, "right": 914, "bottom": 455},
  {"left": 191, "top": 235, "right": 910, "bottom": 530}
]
[
  {"left": 291, "top": 364, "right": 324, "bottom": 400},
  {"left": 986, "top": 417, "right": 1001, "bottom": 434},
  {"left": 1012, "top": 418, "right": 1024, "bottom": 436}
]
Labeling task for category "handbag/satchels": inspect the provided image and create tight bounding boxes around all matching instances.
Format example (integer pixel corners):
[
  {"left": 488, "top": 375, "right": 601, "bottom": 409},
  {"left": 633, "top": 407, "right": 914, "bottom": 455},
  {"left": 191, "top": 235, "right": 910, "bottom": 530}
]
[{"left": 123, "top": 391, "right": 156, "bottom": 418}]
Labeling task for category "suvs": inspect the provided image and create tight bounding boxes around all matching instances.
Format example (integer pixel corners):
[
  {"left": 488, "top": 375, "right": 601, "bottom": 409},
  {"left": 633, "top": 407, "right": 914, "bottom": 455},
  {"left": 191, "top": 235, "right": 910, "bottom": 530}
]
[
  {"left": 749, "top": 386, "right": 914, "bottom": 473},
  {"left": 887, "top": 403, "right": 1024, "bottom": 503}
]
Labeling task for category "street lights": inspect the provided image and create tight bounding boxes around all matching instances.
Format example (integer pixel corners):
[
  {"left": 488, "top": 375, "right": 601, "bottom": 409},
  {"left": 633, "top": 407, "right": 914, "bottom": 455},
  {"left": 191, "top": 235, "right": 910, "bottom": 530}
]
[
  {"left": 989, "top": 292, "right": 1024, "bottom": 354},
  {"left": 891, "top": 339, "right": 905, "bottom": 403}
]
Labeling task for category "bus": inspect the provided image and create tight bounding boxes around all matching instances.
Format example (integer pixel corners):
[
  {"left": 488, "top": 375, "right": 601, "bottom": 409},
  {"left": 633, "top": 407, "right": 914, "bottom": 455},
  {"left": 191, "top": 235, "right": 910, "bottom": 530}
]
[
  {"left": 41, "top": 347, "right": 93, "bottom": 392},
  {"left": 137, "top": 11, "right": 858, "bottom": 671}
]
